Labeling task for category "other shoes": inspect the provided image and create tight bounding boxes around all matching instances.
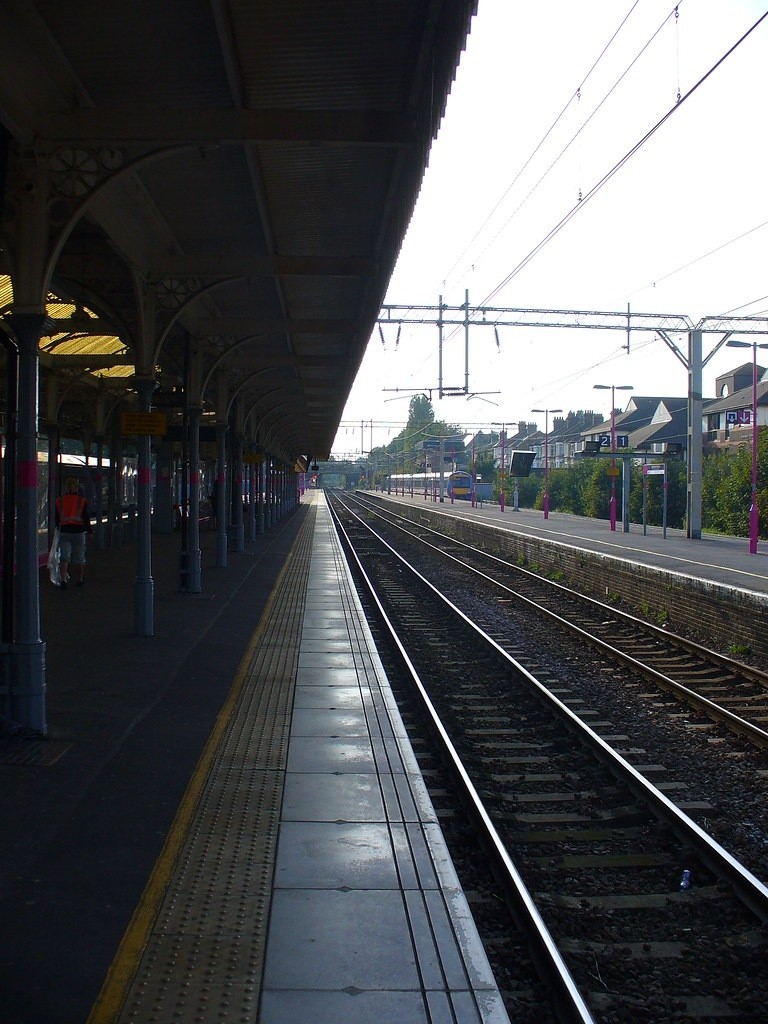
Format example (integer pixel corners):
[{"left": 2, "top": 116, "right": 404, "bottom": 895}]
[
  {"left": 60, "top": 583, "right": 67, "bottom": 589},
  {"left": 77, "top": 579, "right": 84, "bottom": 586}
]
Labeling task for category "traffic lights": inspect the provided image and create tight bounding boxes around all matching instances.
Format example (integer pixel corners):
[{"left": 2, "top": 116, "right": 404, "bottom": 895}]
[
  {"left": 314, "top": 472, "right": 318, "bottom": 479},
  {"left": 361, "top": 472, "right": 365, "bottom": 479}
]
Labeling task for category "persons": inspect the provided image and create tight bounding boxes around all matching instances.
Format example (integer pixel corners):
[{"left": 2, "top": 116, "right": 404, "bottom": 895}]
[
  {"left": 211, "top": 479, "right": 218, "bottom": 528},
  {"left": 55, "top": 477, "right": 93, "bottom": 587}
]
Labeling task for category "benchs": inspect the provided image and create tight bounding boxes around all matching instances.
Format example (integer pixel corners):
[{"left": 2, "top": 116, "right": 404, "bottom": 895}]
[{"left": 173, "top": 500, "right": 209, "bottom": 532}]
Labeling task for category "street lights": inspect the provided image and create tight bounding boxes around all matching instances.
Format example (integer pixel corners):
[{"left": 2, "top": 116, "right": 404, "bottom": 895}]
[
  {"left": 726, "top": 339, "right": 768, "bottom": 552},
  {"left": 593, "top": 384, "right": 633, "bottom": 531},
  {"left": 492, "top": 422, "right": 517, "bottom": 511},
  {"left": 466, "top": 432, "right": 484, "bottom": 506},
  {"left": 531, "top": 409, "right": 563, "bottom": 519}
]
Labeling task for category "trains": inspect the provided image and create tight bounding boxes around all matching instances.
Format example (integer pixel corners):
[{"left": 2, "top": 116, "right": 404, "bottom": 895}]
[{"left": 386, "top": 470, "right": 472, "bottom": 501}]
